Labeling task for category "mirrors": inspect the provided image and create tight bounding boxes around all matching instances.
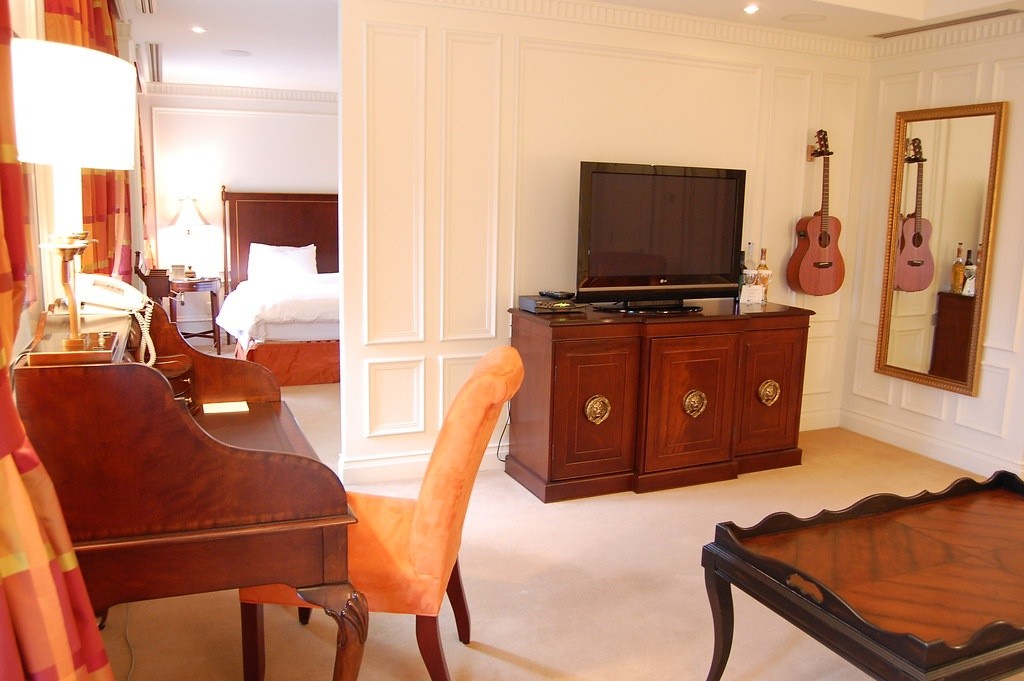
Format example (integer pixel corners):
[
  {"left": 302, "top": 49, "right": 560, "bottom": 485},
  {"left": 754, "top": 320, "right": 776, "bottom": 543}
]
[{"left": 874, "top": 100, "right": 1010, "bottom": 397}]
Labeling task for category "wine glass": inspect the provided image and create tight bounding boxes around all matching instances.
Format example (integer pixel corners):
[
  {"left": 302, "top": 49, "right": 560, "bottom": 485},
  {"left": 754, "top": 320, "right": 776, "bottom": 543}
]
[
  {"left": 965, "top": 266, "right": 975, "bottom": 296},
  {"left": 742, "top": 269, "right": 773, "bottom": 305}
]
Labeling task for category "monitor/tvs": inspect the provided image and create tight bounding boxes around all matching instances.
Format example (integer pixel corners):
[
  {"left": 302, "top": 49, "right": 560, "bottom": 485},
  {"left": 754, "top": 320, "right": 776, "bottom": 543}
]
[{"left": 574, "top": 161, "right": 746, "bottom": 316}]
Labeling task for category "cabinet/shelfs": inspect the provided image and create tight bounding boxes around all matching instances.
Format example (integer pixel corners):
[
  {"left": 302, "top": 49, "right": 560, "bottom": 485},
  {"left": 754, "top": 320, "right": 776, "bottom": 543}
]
[
  {"left": 928, "top": 289, "right": 975, "bottom": 382},
  {"left": 505, "top": 298, "right": 816, "bottom": 503}
]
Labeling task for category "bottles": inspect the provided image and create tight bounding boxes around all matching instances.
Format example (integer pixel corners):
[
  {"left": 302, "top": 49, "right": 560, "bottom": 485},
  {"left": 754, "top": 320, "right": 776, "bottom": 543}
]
[
  {"left": 964, "top": 250, "right": 973, "bottom": 292},
  {"left": 745, "top": 241, "right": 756, "bottom": 285},
  {"left": 756, "top": 247, "right": 769, "bottom": 303},
  {"left": 734, "top": 251, "right": 746, "bottom": 302},
  {"left": 951, "top": 242, "right": 965, "bottom": 292}
]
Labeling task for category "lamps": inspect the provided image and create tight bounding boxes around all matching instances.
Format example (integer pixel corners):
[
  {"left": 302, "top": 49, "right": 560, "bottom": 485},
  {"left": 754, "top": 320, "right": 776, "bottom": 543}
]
[
  {"left": 166, "top": 198, "right": 211, "bottom": 277},
  {"left": 8, "top": 38, "right": 143, "bottom": 321}
]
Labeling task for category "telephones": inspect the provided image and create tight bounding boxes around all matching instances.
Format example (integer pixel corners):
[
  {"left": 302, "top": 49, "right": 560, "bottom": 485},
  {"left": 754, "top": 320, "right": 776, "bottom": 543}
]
[{"left": 73, "top": 271, "right": 148, "bottom": 312}]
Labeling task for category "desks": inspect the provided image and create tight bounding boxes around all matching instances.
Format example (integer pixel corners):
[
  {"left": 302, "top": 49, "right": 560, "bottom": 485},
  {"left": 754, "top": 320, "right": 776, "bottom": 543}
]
[
  {"left": 169, "top": 277, "right": 222, "bottom": 355},
  {"left": 13, "top": 300, "right": 359, "bottom": 681},
  {"left": 701, "top": 470, "right": 1024, "bottom": 681}
]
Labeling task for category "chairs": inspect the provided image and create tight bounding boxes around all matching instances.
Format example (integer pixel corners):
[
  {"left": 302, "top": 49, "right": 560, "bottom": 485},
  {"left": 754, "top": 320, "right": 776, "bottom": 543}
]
[{"left": 238, "top": 345, "right": 524, "bottom": 680}]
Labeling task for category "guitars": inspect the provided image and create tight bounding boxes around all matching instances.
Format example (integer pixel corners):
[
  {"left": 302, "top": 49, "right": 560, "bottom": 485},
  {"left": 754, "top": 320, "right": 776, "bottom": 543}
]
[
  {"left": 895, "top": 137, "right": 935, "bottom": 293},
  {"left": 786, "top": 129, "right": 846, "bottom": 296}
]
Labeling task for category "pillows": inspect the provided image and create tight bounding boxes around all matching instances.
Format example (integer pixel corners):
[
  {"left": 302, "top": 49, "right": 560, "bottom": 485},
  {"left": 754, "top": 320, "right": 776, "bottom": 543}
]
[{"left": 247, "top": 242, "right": 318, "bottom": 286}]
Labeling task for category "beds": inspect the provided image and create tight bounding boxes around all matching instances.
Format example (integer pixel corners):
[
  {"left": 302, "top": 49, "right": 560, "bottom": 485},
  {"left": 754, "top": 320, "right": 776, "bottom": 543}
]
[{"left": 215, "top": 185, "right": 340, "bottom": 387}]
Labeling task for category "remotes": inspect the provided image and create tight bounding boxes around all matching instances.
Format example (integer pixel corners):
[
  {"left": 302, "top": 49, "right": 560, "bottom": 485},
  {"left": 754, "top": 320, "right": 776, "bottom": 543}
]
[{"left": 539, "top": 290, "right": 575, "bottom": 301}]
